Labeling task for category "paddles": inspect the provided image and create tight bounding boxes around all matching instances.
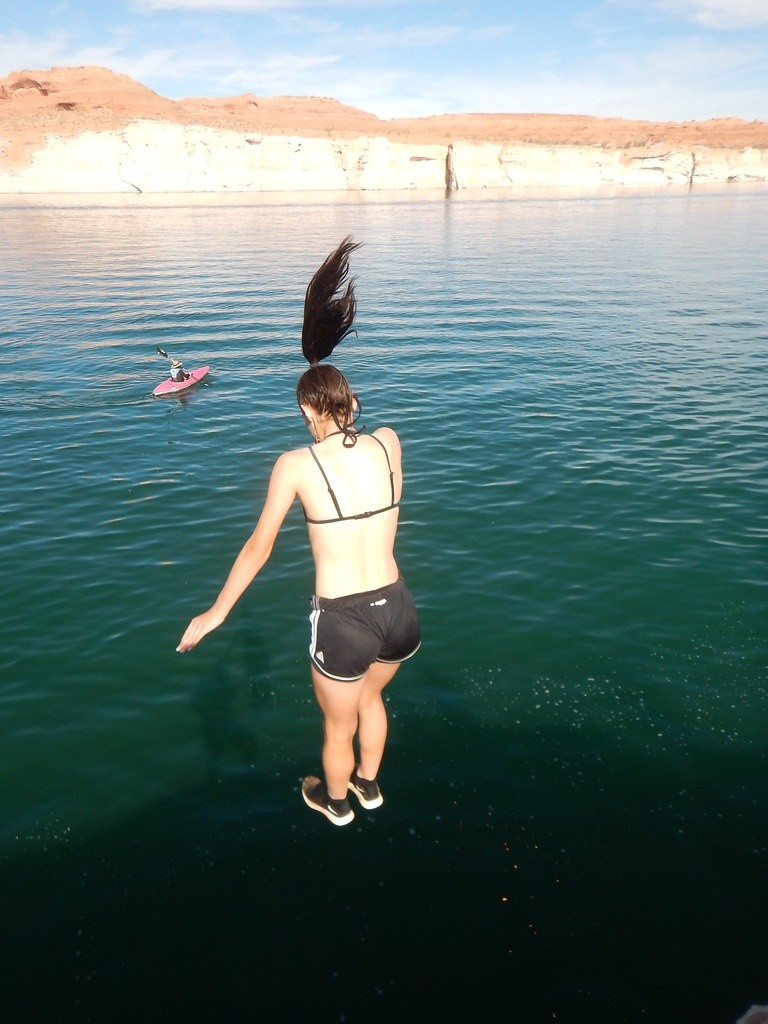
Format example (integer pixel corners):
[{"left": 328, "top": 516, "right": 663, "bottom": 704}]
[{"left": 157, "top": 346, "right": 204, "bottom": 383}]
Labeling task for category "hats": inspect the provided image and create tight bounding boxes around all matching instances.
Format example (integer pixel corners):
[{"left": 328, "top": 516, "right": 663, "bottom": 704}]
[{"left": 171, "top": 361, "right": 183, "bottom": 368}]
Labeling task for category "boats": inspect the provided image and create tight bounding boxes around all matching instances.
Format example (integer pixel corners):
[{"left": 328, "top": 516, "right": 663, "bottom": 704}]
[{"left": 153, "top": 366, "right": 210, "bottom": 396}]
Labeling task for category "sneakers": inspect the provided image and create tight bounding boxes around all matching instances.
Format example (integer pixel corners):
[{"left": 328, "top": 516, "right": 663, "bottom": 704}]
[
  {"left": 348, "top": 763, "right": 383, "bottom": 810},
  {"left": 302, "top": 775, "right": 354, "bottom": 826}
]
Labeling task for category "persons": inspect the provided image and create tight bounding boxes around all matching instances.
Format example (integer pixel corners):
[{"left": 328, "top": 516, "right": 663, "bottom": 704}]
[
  {"left": 176, "top": 232, "right": 422, "bottom": 825},
  {"left": 170, "top": 361, "right": 191, "bottom": 382}
]
